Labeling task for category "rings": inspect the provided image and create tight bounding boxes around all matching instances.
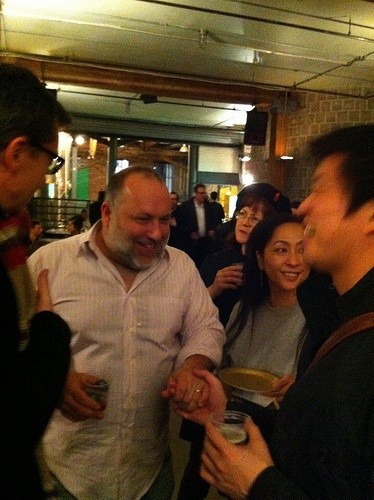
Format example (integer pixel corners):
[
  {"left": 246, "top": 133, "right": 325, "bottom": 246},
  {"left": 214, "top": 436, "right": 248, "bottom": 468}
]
[{"left": 195, "top": 389, "right": 202, "bottom": 394}]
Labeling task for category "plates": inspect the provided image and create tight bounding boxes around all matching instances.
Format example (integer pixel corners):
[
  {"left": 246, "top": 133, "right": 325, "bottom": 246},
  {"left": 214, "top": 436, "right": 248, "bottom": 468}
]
[{"left": 220, "top": 365, "right": 281, "bottom": 394}]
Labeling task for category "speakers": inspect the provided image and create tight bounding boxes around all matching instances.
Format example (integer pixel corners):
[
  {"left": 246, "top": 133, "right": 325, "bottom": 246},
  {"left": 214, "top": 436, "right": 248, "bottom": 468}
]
[{"left": 244, "top": 110, "right": 269, "bottom": 145}]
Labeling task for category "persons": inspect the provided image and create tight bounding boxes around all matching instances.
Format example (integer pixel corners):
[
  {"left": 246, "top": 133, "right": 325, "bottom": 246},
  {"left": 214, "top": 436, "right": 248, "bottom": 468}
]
[
  {"left": 173, "top": 181, "right": 292, "bottom": 499},
  {"left": 166, "top": 190, "right": 180, "bottom": 247},
  {"left": 25, "top": 220, "right": 45, "bottom": 258},
  {"left": 164, "top": 122, "right": 374, "bottom": 499},
  {"left": 208, "top": 192, "right": 225, "bottom": 224},
  {"left": 79, "top": 209, "right": 91, "bottom": 232},
  {"left": 65, "top": 216, "right": 84, "bottom": 238},
  {"left": 89, "top": 191, "right": 105, "bottom": 226},
  {"left": 177, "top": 182, "right": 220, "bottom": 242},
  {"left": 0, "top": 61, "right": 72, "bottom": 500},
  {"left": 27, "top": 163, "right": 227, "bottom": 500},
  {"left": 176, "top": 209, "right": 310, "bottom": 500}
]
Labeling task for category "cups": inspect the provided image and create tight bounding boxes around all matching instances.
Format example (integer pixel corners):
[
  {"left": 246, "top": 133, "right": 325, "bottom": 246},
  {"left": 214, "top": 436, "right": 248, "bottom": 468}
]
[
  {"left": 210, "top": 410, "right": 249, "bottom": 498},
  {"left": 86, "top": 378, "right": 108, "bottom": 423}
]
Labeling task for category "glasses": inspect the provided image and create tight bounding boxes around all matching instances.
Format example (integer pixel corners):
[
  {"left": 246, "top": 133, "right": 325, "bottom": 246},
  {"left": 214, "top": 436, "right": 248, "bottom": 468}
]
[
  {"left": 233, "top": 210, "right": 263, "bottom": 227},
  {"left": 30, "top": 141, "right": 65, "bottom": 174},
  {"left": 197, "top": 191, "right": 206, "bottom": 195}
]
